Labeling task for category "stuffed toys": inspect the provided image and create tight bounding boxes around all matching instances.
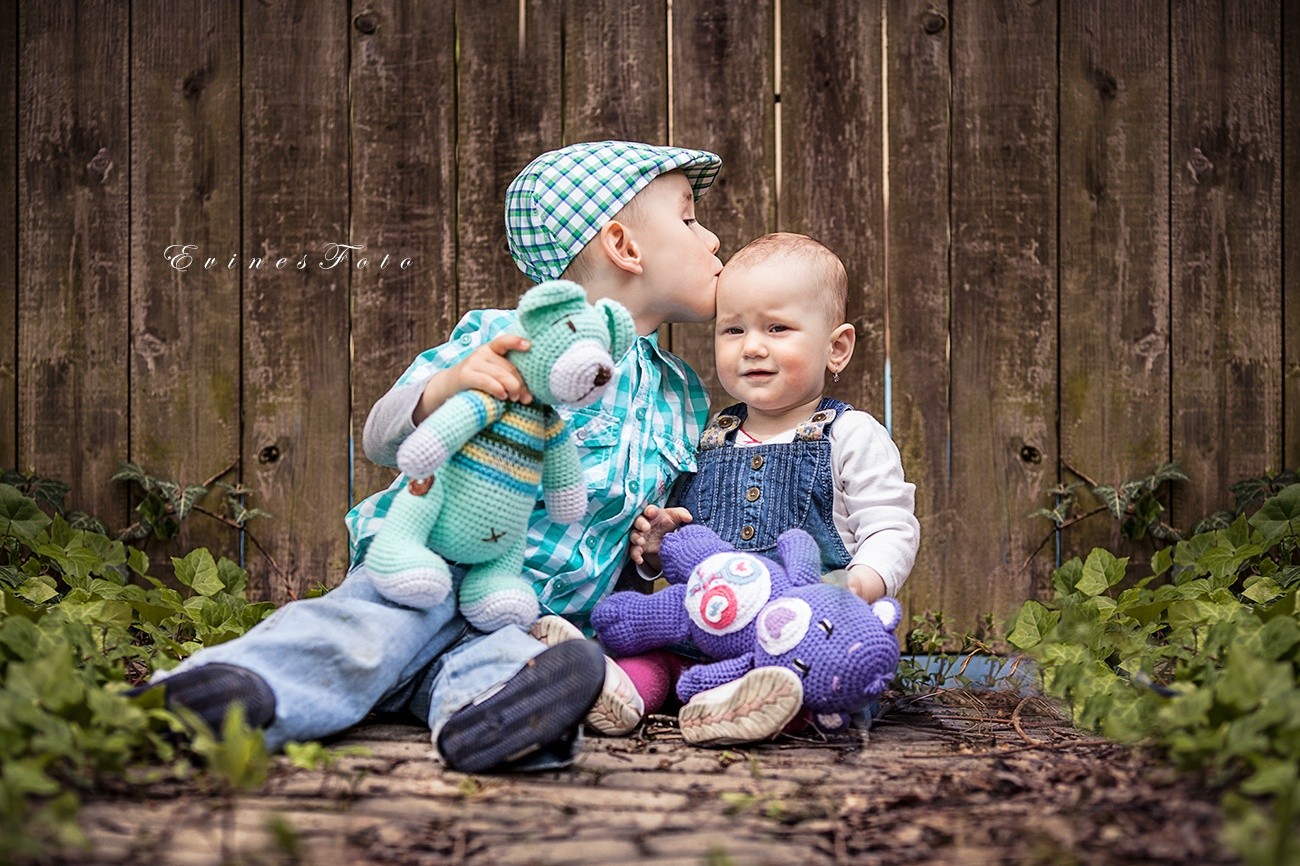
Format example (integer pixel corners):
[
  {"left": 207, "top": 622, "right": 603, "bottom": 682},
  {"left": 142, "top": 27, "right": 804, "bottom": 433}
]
[
  {"left": 363, "top": 280, "right": 642, "bottom": 635},
  {"left": 590, "top": 526, "right": 903, "bottom": 735}
]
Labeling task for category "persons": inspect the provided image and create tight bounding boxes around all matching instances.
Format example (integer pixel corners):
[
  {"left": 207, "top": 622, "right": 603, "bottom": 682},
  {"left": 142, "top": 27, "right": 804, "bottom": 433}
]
[
  {"left": 119, "top": 141, "right": 725, "bottom": 776},
  {"left": 534, "top": 232, "right": 921, "bottom": 751}
]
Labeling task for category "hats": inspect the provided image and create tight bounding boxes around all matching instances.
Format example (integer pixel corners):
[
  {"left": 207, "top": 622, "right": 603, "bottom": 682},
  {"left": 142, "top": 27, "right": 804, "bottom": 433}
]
[{"left": 505, "top": 140, "right": 724, "bottom": 284}]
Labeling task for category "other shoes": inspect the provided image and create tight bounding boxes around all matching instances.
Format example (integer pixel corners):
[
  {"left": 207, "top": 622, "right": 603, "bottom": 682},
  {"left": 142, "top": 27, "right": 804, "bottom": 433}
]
[
  {"left": 437, "top": 639, "right": 605, "bottom": 775},
  {"left": 523, "top": 614, "right": 645, "bottom": 736},
  {"left": 678, "top": 664, "right": 805, "bottom": 747},
  {"left": 105, "top": 660, "right": 277, "bottom": 754}
]
